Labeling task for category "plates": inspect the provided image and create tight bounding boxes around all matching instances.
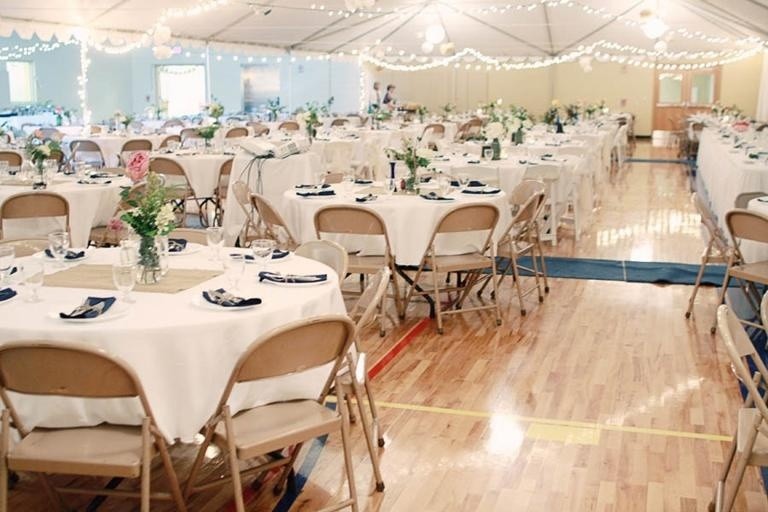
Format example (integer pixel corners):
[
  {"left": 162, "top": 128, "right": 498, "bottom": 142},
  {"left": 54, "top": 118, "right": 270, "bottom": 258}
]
[
  {"left": 256, "top": 269, "right": 333, "bottom": 286},
  {"left": 233, "top": 247, "right": 295, "bottom": 263},
  {"left": 167, "top": 242, "right": 202, "bottom": 255},
  {"left": 50, "top": 303, "right": 131, "bottom": 322},
  {"left": 2, "top": 165, "right": 120, "bottom": 191},
  {"left": 191, "top": 289, "right": 262, "bottom": 310},
  {"left": 294, "top": 177, "right": 499, "bottom": 206},
  {"left": 114, "top": 254, "right": 134, "bottom": 305},
  {"left": 43, "top": 248, "right": 89, "bottom": 261}
]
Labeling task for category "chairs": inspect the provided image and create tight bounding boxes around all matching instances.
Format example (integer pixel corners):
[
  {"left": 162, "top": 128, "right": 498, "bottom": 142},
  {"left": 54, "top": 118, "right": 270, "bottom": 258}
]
[
  {"left": 666, "top": 117, "right": 708, "bottom": 161},
  {"left": 685, "top": 193, "right": 768, "bottom": 511}
]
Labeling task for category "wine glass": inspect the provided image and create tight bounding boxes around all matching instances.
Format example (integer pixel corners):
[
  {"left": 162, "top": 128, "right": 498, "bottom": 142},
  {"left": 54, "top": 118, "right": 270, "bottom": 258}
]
[
  {"left": 254, "top": 241, "right": 273, "bottom": 282},
  {"left": 74, "top": 163, "right": 83, "bottom": 183},
  {"left": 225, "top": 254, "right": 244, "bottom": 298},
  {"left": 0, "top": 161, "right": 7, "bottom": 180},
  {"left": 385, "top": 180, "right": 392, "bottom": 199},
  {"left": 0, "top": 244, "right": 16, "bottom": 291},
  {"left": 315, "top": 171, "right": 325, "bottom": 189},
  {"left": 25, "top": 260, "right": 44, "bottom": 304},
  {"left": 47, "top": 161, "right": 58, "bottom": 185},
  {"left": 207, "top": 226, "right": 223, "bottom": 260},
  {"left": 483, "top": 149, "right": 494, "bottom": 165},
  {"left": 50, "top": 233, "right": 66, "bottom": 269},
  {"left": 439, "top": 176, "right": 450, "bottom": 202}
]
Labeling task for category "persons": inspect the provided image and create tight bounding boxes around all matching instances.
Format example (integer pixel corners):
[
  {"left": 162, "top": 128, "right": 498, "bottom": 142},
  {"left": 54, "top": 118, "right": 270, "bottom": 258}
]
[
  {"left": 383, "top": 84, "right": 397, "bottom": 104},
  {"left": 367, "top": 80, "right": 382, "bottom": 113}
]
